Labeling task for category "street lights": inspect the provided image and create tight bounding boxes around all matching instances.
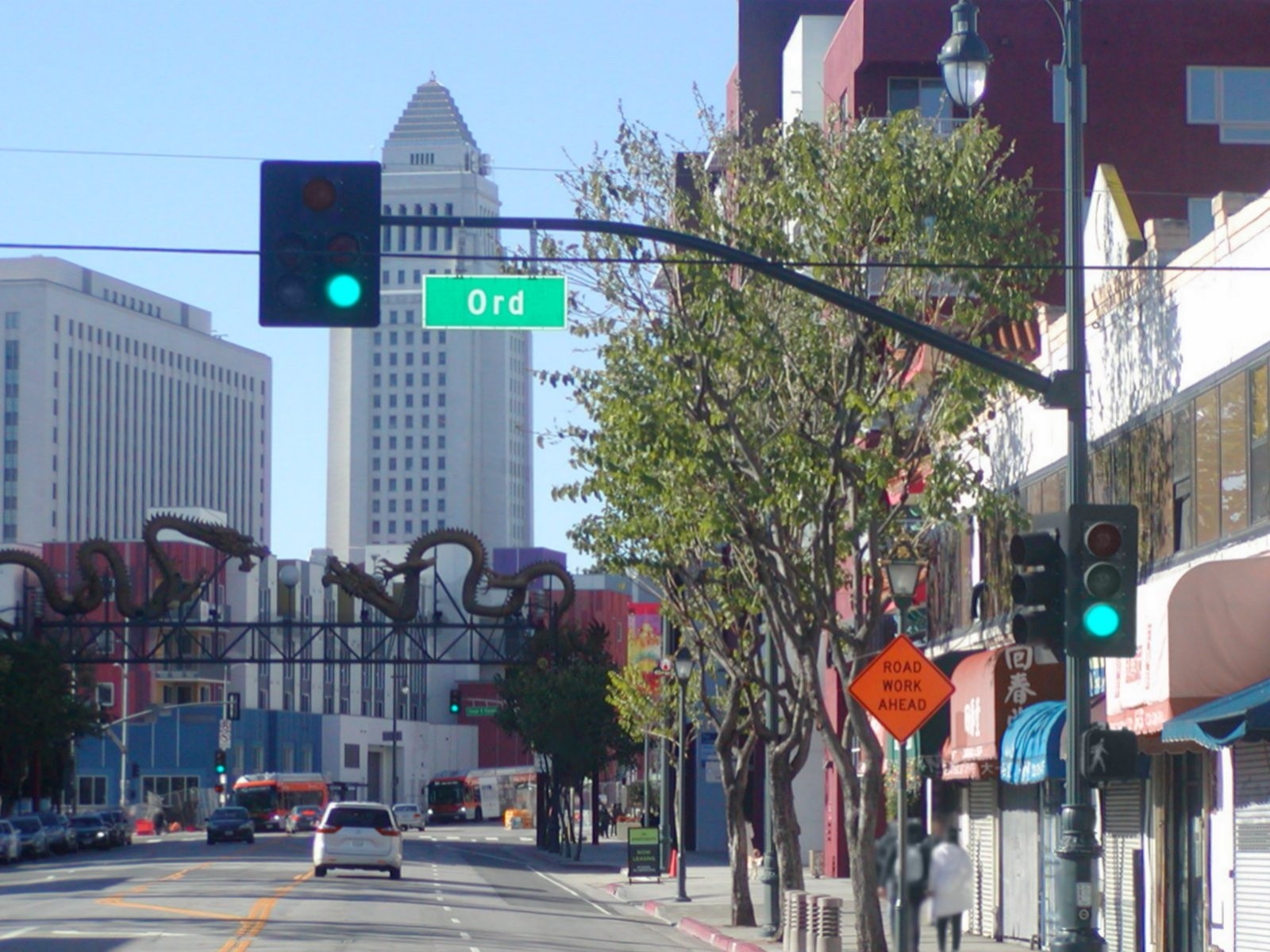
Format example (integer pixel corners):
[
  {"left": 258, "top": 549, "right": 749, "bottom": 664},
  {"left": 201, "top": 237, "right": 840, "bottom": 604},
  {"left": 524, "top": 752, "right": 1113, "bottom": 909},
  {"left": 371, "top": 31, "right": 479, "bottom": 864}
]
[
  {"left": 671, "top": 647, "right": 693, "bottom": 901},
  {"left": 936, "top": 0, "right": 1108, "bottom": 952},
  {"left": 878, "top": 537, "right": 925, "bottom": 952},
  {"left": 111, "top": 618, "right": 130, "bottom": 807},
  {"left": 390, "top": 656, "right": 410, "bottom": 805}
]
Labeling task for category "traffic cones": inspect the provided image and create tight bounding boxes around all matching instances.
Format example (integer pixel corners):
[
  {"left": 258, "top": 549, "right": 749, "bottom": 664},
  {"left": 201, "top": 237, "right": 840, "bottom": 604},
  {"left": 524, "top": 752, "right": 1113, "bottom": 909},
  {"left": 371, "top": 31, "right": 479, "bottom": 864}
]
[{"left": 668, "top": 850, "right": 678, "bottom": 877}]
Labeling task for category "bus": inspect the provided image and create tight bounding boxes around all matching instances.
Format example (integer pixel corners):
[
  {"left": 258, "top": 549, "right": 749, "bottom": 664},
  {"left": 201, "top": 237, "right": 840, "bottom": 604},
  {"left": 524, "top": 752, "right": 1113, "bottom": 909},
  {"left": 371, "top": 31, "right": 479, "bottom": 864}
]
[
  {"left": 422, "top": 766, "right": 536, "bottom": 822},
  {"left": 229, "top": 772, "right": 328, "bottom": 827}
]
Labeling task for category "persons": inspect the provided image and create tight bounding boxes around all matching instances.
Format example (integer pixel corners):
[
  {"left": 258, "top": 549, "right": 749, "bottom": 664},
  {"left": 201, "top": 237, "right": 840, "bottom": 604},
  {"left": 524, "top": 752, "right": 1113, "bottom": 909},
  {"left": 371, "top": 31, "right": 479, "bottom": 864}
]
[
  {"left": 926, "top": 826, "right": 973, "bottom": 952},
  {"left": 600, "top": 803, "right": 619, "bottom": 838},
  {"left": 151, "top": 807, "right": 167, "bottom": 836},
  {"left": 871, "top": 812, "right": 929, "bottom": 952}
]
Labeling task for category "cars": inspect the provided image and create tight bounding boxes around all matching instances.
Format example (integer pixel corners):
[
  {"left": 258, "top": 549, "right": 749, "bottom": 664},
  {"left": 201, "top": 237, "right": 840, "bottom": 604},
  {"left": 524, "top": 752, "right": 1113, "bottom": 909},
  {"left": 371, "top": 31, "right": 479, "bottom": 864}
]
[
  {"left": 313, "top": 801, "right": 407, "bottom": 881},
  {"left": 0, "top": 811, "right": 131, "bottom": 863},
  {"left": 285, "top": 804, "right": 320, "bottom": 833},
  {"left": 204, "top": 807, "right": 255, "bottom": 845},
  {"left": 393, "top": 803, "right": 424, "bottom": 832}
]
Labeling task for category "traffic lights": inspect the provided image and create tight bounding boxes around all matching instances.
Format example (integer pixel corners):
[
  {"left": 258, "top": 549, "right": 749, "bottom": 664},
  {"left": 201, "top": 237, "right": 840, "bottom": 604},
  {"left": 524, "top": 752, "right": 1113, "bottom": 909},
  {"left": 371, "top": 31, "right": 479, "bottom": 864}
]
[
  {"left": 1067, "top": 503, "right": 1138, "bottom": 659},
  {"left": 216, "top": 751, "right": 226, "bottom": 774},
  {"left": 449, "top": 690, "right": 461, "bottom": 715},
  {"left": 1079, "top": 729, "right": 1136, "bottom": 783},
  {"left": 213, "top": 785, "right": 223, "bottom": 793},
  {"left": 1010, "top": 513, "right": 1065, "bottom": 649},
  {"left": 258, "top": 158, "right": 382, "bottom": 328}
]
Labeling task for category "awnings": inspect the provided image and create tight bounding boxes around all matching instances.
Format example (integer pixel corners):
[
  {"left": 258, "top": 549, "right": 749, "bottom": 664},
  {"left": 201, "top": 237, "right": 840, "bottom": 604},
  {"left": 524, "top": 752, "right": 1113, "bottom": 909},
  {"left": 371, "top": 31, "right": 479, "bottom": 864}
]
[
  {"left": 1161, "top": 679, "right": 1270, "bottom": 751},
  {"left": 1059, "top": 692, "right": 1152, "bottom": 761},
  {"left": 950, "top": 643, "right": 1066, "bottom": 764},
  {"left": 1105, "top": 557, "right": 1270, "bottom": 754},
  {"left": 858, "top": 650, "right": 985, "bottom": 777},
  {"left": 941, "top": 739, "right": 999, "bottom": 781},
  {"left": 998, "top": 700, "right": 1149, "bottom": 785}
]
